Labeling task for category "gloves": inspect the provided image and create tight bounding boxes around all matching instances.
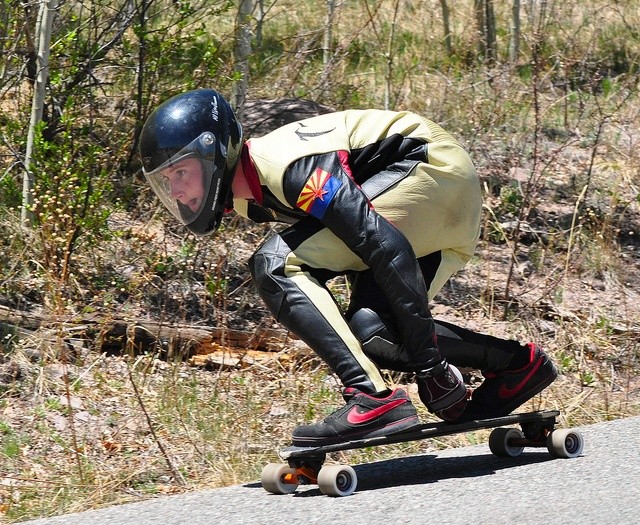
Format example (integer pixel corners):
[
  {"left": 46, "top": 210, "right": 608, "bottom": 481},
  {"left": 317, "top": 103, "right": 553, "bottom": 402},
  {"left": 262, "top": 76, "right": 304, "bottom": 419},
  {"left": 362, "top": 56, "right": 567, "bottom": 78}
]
[{"left": 403, "top": 332, "right": 471, "bottom": 424}]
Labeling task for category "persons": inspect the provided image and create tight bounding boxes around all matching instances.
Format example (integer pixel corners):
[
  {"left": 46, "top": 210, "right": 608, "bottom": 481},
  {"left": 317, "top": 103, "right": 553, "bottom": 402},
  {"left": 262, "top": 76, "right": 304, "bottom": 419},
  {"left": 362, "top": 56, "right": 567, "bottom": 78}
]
[{"left": 138, "top": 88, "right": 559, "bottom": 448}]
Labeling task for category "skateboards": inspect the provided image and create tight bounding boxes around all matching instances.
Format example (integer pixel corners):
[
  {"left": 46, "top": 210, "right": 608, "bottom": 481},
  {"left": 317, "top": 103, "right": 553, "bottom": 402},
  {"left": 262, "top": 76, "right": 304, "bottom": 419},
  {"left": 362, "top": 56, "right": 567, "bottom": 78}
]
[{"left": 261, "top": 409, "right": 582, "bottom": 496}]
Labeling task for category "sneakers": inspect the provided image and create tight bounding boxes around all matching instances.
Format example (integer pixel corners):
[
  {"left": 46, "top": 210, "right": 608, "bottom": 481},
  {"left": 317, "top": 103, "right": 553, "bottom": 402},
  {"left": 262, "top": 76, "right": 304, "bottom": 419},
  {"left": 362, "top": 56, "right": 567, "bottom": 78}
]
[
  {"left": 291, "top": 387, "right": 422, "bottom": 447},
  {"left": 472, "top": 343, "right": 558, "bottom": 419}
]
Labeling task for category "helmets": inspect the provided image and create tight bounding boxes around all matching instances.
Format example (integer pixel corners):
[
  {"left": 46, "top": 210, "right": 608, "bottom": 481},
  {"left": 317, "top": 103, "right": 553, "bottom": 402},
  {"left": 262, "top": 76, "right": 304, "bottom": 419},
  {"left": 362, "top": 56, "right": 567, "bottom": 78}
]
[{"left": 138, "top": 87, "right": 244, "bottom": 237}]
